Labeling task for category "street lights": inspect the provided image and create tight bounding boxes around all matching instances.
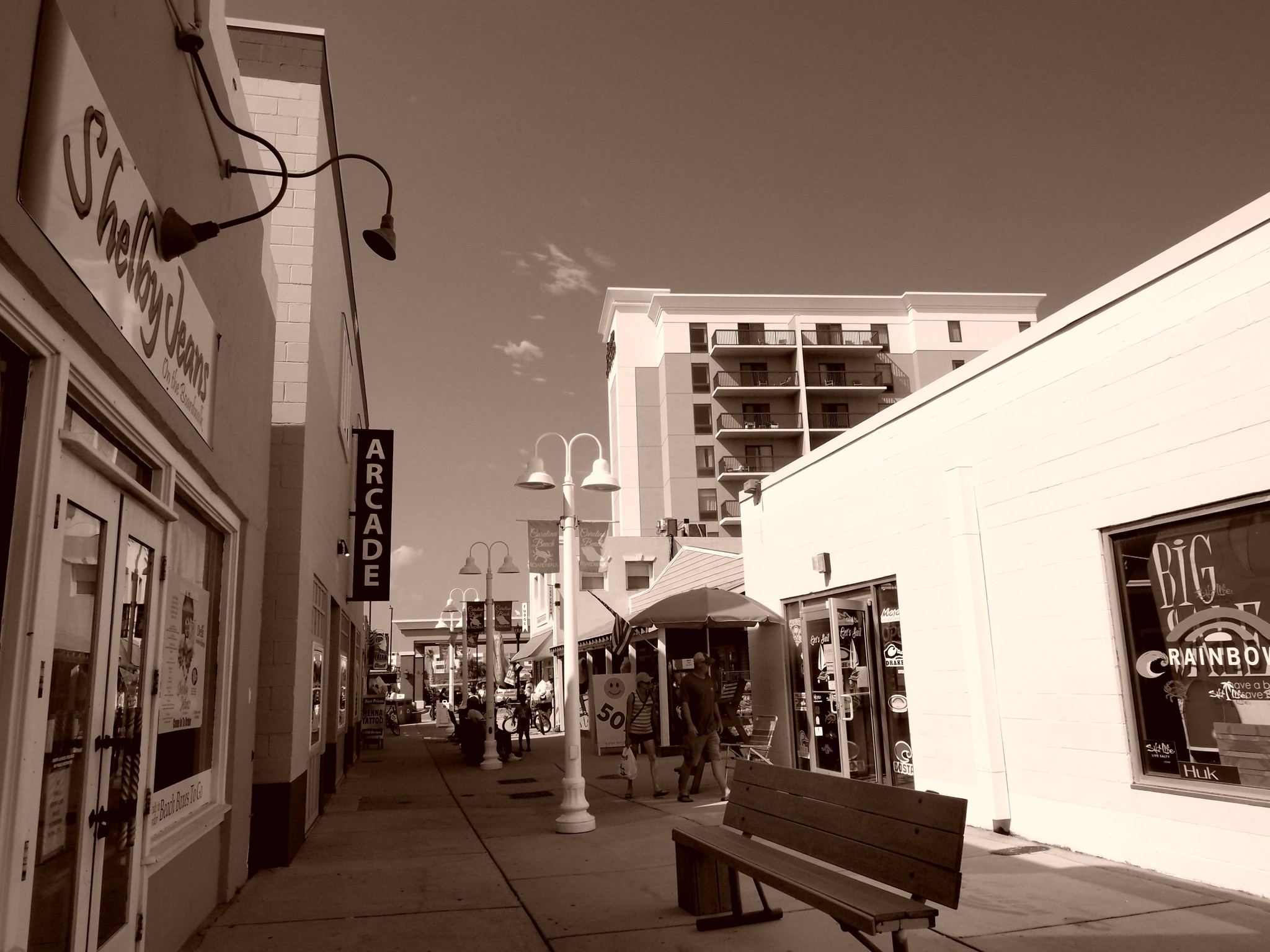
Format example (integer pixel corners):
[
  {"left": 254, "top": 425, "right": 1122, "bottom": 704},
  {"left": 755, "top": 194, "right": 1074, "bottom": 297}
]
[
  {"left": 514, "top": 432, "right": 622, "bottom": 833},
  {"left": 389, "top": 607, "right": 394, "bottom": 666},
  {"left": 434, "top": 610, "right": 463, "bottom": 734},
  {"left": 442, "top": 589, "right": 481, "bottom": 709},
  {"left": 458, "top": 541, "right": 520, "bottom": 770}
]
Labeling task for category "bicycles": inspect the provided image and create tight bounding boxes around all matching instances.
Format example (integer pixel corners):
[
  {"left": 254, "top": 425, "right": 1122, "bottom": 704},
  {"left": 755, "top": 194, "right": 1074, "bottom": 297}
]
[
  {"left": 530, "top": 700, "right": 551, "bottom": 735},
  {"left": 500, "top": 696, "right": 519, "bottom": 734},
  {"left": 386, "top": 705, "right": 400, "bottom": 737}
]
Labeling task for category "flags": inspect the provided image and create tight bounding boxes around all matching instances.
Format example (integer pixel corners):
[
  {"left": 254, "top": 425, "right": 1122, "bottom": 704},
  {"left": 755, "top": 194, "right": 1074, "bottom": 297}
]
[
  {"left": 504, "top": 662, "right": 524, "bottom": 688},
  {"left": 588, "top": 590, "right": 635, "bottom": 655}
]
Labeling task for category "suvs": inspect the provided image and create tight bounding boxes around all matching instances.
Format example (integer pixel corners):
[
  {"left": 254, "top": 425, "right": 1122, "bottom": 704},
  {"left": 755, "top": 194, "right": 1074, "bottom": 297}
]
[
  {"left": 423, "top": 681, "right": 434, "bottom": 706},
  {"left": 495, "top": 682, "right": 524, "bottom": 707},
  {"left": 476, "top": 679, "right": 496, "bottom": 694}
]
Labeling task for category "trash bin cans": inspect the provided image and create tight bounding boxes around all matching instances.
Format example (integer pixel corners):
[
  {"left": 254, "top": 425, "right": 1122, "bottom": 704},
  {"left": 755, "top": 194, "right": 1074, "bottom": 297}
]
[{"left": 395, "top": 698, "right": 412, "bottom": 725}]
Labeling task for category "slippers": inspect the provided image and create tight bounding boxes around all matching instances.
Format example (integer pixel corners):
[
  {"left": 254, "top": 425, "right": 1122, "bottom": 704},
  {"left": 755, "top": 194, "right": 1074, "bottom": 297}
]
[
  {"left": 721, "top": 793, "right": 731, "bottom": 801},
  {"left": 653, "top": 790, "right": 669, "bottom": 798},
  {"left": 625, "top": 794, "right": 633, "bottom": 799},
  {"left": 677, "top": 795, "right": 693, "bottom": 802}
]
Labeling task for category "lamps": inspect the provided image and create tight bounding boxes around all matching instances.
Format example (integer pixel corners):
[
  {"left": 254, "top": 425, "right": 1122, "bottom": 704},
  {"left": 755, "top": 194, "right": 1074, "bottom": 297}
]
[
  {"left": 812, "top": 553, "right": 831, "bottom": 573},
  {"left": 337, "top": 539, "right": 350, "bottom": 557},
  {"left": 160, "top": 34, "right": 288, "bottom": 262},
  {"left": 224, "top": 154, "right": 396, "bottom": 261}
]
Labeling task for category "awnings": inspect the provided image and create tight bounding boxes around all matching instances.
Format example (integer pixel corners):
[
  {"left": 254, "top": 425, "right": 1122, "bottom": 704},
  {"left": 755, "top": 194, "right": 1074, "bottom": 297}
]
[{"left": 510, "top": 630, "right": 553, "bottom": 662}]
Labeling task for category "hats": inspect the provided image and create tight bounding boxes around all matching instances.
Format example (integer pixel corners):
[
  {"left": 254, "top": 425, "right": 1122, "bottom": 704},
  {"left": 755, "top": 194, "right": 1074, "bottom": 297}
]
[
  {"left": 548, "top": 674, "right": 554, "bottom": 679},
  {"left": 636, "top": 672, "right": 654, "bottom": 684},
  {"left": 693, "top": 652, "right": 713, "bottom": 664}
]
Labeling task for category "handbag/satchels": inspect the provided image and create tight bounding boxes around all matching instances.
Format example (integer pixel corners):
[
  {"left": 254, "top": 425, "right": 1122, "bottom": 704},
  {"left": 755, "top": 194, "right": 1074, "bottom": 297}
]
[{"left": 618, "top": 746, "right": 637, "bottom": 779}]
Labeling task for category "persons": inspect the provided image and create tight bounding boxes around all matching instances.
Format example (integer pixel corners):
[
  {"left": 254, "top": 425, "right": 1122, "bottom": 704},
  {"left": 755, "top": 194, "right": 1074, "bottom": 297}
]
[
  {"left": 439, "top": 688, "right": 449, "bottom": 703},
  {"left": 477, "top": 683, "right": 486, "bottom": 704},
  {"left": 677, "top": 652, "right": 730, "bottom": 802},
  {"left": 512, "top": 693, "right": 533, "bottom": 752},
  {"left": 624, "top": 672, "right": 669, "bottom": 799},
  {"left": 467, "top": 696, "right": 521, "bottom": 761},
  {"left": 543, "top": 675, "right": 554, "bottom": 729},
  {"left": 390, "top": 688, "right": 396, "bottom": 699},
  {"left": 469, "top": 686, "right": 482, "bottom": 704}
]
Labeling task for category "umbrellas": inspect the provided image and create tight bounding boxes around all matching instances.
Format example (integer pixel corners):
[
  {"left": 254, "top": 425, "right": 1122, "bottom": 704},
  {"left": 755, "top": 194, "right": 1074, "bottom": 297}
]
[{"left": 628, "top": 585, "right": 787, "bottom": 677}]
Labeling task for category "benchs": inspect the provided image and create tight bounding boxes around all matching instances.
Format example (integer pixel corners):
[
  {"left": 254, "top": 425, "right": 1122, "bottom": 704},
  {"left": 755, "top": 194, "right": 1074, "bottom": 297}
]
[
  {"left": 779, "top": 339, "right": 787, "bottom": 344},
  {"left": 671, "top": 759, "right": 968, "bottom": 952}
]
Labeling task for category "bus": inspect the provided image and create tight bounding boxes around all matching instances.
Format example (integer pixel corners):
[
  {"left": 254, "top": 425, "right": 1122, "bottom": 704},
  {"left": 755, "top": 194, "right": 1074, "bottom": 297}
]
[{"left": 430, "top": 682, "right": 472, "bottom": 693}]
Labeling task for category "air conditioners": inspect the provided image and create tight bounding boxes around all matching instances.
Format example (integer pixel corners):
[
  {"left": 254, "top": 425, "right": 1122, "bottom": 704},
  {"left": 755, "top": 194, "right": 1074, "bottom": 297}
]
[{"left": 682, "top": 523, "right": 708, "bottom": 537}]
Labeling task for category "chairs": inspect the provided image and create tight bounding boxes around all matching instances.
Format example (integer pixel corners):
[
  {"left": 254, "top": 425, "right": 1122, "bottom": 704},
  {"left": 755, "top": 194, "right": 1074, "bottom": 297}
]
[
  {"left": 725, "top": 465, "right": 749, "bottom": 472},
  {"left": 744, "top": 421, "right": 778, "bottom": 428},
  {"left": 720, "top": 715, "right": 778, "bottom": 783},
  {"left": 758, "top": 339, "right": 764, "bottom": 345},
  {"left": 757, "top": 377, "right": 791, "bottom": 386},
  {"left": 853, "top": 380, "right": 862, "bottom": 386},
  {"left": 824, "top": 379, "right": 833, "bottom": 386},
  {"left": 845, "top": 340, "right": 873, "bottom": 345},
  {"left": 721, "top": 679, "right": 750, "bottom": 757}
]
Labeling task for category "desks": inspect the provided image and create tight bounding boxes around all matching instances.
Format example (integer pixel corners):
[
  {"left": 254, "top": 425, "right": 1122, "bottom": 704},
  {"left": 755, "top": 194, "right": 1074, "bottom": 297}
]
[{"left": 758, "top": 425, "right": 766, "bottom": 428}]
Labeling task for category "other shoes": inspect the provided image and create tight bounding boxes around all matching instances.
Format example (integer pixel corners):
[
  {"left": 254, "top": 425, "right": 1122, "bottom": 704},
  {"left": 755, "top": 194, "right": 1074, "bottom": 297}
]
[{"left": 508, "top": 752, "right": 522, "bottom": 761}]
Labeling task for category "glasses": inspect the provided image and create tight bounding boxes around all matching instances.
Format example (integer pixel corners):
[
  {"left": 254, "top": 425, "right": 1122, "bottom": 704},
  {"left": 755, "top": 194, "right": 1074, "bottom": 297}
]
[{"left": 702, "top": 661, "right": 710, "bottom": 666}]
